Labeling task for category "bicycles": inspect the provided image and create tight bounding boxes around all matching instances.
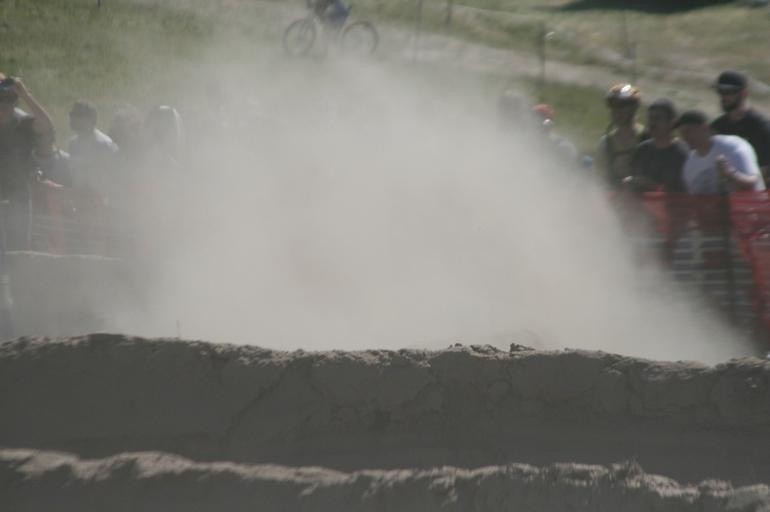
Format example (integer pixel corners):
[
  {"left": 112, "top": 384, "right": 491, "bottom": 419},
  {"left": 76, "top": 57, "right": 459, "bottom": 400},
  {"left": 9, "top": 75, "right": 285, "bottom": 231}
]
[{"left": 282, "top": 3, "right": 379, "bottom": 60}]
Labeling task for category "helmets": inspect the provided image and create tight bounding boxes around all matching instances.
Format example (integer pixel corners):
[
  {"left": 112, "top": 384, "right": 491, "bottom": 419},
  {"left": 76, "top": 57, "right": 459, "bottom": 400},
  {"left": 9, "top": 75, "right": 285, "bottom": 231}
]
[{"left": 603, "top": 83, "right": 641, "bottom": 104}]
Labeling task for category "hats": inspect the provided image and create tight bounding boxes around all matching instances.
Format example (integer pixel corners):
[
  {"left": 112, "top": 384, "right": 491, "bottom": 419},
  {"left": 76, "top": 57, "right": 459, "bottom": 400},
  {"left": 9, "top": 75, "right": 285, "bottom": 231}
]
[
  {"left": 711, "top": 70, "right": 750, "bottom": 91},
  {"left": 671, "top": 109, "right": 708, "bottom": 129}
]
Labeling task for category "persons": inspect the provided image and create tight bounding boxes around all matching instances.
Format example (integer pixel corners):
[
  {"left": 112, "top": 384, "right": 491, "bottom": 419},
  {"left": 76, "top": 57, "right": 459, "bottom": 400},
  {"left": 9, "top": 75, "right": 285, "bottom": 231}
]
[
  {"left": 493, "top": 67, "right": 770, "bottom": 191},
  {"left": 1, "top": 72, "right": 180, "bottom": 220},
  {"left": 304, "top": 0, "right": 350, "bottom": 38}
]
